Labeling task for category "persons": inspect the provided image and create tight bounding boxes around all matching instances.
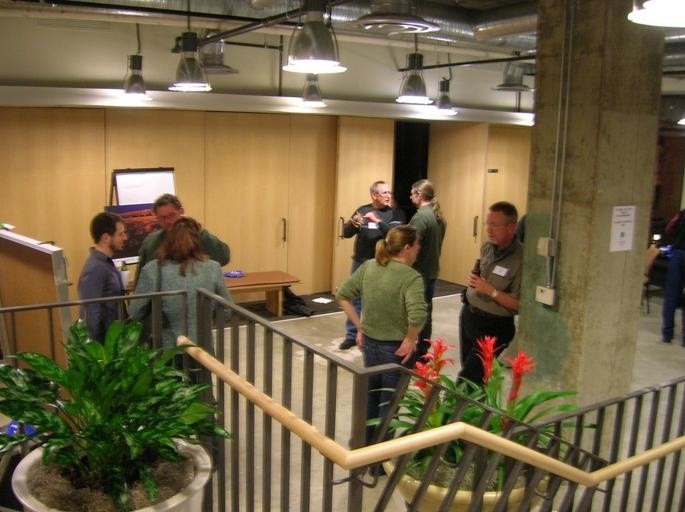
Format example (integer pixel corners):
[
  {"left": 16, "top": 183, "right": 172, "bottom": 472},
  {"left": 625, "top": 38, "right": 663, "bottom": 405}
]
[
  {"left": 335, "top": 224, "right": 428, "bottom": 477},
  {"left": 127, "top": 217, "right": 234, "bottom": 471},
  {"left": 134, "top": 194, "right": 230, "bottom": 294},
  {"left": 456, "top": 201, "right": 525, "bottom": 399},
  {"left": 339, "top": 181, "right": 406, "bottom": 350},
  {"left": 657, "top": 210, "right": 685, "bottom": 347},
  {"left": 77, "top": 213, "right": 128, "bottom": 345},
  {"left": 406, "top": 179, "right": 448, "bottom": 367}
]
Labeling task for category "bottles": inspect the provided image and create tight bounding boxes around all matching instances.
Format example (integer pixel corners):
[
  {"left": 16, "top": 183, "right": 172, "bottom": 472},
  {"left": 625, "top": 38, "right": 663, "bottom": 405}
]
[{"left": 469, "top": 259, "right": 481, "bottom": 288}]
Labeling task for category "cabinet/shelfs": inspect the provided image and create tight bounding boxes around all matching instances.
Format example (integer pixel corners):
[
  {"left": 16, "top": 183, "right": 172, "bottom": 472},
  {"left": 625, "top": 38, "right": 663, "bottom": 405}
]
[{"left": 648, "top": 120, "right": 684, "bottom": 247}]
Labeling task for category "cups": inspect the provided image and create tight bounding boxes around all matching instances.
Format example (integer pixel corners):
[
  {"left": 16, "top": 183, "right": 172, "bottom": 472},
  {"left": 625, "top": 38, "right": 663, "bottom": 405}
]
[{"left": 119, "top": 261, "right": 130, "bottom": 290}]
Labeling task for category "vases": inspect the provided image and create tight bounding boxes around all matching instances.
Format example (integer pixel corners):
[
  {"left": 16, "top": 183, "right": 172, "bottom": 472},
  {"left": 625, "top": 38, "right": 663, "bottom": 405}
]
[{"left": 378, "top": 447, "right": 574, "bottom": 511}]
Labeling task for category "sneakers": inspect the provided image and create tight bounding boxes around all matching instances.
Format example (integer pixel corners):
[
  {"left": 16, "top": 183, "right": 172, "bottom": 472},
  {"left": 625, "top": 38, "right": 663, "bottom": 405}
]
[{"left": 339, "top": 340, "right": 356, "bottom": 350}]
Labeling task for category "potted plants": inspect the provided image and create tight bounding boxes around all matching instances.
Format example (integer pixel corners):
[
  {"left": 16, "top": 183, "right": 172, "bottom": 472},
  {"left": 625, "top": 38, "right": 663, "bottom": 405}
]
[{"left": 0, "top": 315, "right": 235, "bottom": 512}]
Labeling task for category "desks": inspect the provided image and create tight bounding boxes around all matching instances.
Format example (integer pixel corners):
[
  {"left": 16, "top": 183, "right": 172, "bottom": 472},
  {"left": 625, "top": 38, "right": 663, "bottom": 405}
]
[{"left": 222, "top": 270, "right": 301, "bottom": 317}]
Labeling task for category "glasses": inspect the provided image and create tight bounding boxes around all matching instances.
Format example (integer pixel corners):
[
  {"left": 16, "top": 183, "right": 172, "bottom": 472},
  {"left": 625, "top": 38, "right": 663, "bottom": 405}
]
[{"left": 159, "top": 212, "right": 177, "bottom": 220}]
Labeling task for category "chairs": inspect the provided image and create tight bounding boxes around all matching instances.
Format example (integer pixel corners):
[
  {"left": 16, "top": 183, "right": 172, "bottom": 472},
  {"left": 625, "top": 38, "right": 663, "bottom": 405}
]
[{"left": 641, "top": 245, "right": 659, "bottom": 314}]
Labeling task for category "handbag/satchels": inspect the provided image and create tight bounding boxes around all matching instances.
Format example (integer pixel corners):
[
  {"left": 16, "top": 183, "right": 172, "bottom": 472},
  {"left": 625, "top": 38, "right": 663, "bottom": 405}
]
[{"left": 124, "top": 260, "right": 174, "bottom": 348}]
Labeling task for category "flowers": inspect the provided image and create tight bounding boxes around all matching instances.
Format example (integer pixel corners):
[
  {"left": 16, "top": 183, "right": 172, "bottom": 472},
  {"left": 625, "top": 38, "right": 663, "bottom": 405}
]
[{"left": 370, "top": 333, "right": 574, "bottom": 477}]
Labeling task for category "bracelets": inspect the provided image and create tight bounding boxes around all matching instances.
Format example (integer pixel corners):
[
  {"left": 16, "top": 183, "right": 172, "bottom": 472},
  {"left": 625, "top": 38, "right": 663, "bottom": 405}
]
[
  {"left": 408, "top": 336, "right": 420, "bottom": 343},
  {"left": 490, "top": 290, "right": 498, "bottom": 299}
]
